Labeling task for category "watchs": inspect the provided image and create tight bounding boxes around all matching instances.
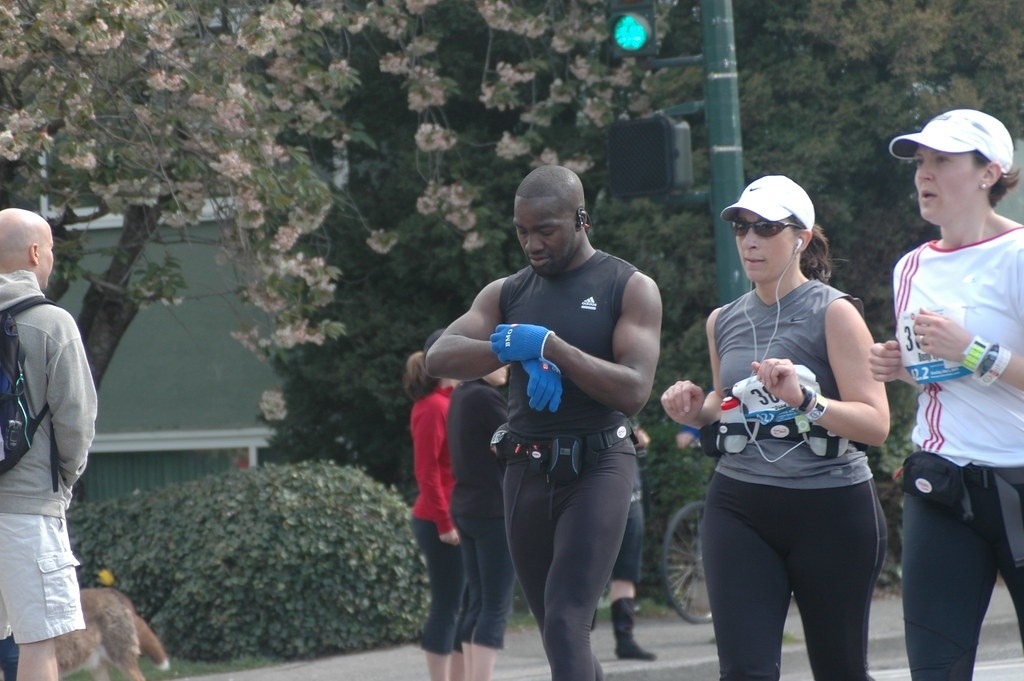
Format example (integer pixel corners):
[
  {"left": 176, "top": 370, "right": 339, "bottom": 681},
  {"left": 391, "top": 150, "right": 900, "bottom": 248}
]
[{"left": 978, "top": 344, "right": 999, "bottom": 377}]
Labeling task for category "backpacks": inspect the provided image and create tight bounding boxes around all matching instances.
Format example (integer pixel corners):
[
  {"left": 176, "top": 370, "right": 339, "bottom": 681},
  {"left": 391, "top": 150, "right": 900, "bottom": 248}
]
[{"left": 0, "top": 299, "right": 56, "bottom": 475}]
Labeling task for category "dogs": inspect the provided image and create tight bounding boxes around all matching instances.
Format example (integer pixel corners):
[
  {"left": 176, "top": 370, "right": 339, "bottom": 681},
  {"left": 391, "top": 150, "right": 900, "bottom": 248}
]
[{"left": 56, "top": 585, "right": 170, "bottom": 681}]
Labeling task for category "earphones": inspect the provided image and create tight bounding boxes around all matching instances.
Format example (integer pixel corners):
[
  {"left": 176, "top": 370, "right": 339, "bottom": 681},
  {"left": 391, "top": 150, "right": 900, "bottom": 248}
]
[
  {"left": 576, "top": 213, "right": 584, "bottom": 228},
  {"left": 793, "top": 238, "right": 803, "bottom": 253}
]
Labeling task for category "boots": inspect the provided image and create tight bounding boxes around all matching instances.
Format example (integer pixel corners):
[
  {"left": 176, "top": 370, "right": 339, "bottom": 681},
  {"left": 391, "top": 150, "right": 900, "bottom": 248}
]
[{"left": 611, "top": 597, "right": 656, "bottom": 661}]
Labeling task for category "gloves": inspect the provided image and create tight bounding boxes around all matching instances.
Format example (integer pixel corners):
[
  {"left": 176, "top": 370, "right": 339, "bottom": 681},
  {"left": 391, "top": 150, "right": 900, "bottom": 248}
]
[
  {"left": 490, "top": 324, "right": 556, "bottom": 362},
  {"left": 522, "top": 358, "right": 561, "bottom": 413}
]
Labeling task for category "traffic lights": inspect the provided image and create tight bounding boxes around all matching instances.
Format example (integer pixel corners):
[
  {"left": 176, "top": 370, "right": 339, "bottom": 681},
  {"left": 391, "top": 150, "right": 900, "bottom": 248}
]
[
  {"left": 609, "top": 0, "right": 658, "bottom": 58},
  {"left": 606, "top": 115, "right": 692, "bottom": 198}
]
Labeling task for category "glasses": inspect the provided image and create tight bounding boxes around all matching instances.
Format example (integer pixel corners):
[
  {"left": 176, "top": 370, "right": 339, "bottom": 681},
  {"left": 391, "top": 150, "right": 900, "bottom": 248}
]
[{"left": 729, "top": 218, "right": 805, "bottom": 239}]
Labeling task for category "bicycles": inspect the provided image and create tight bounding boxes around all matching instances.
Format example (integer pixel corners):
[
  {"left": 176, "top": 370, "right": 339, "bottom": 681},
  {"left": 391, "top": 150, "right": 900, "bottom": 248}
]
[{"left": 660, "top": 501, "right": 713, "bottom": 623}]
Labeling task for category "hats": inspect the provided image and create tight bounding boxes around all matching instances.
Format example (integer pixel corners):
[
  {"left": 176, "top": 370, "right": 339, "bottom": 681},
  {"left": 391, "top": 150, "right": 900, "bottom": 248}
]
[
  {"left": 722, "top": 174, "right": 815, "bottom": 232},
  {"left": 889, "top": 109, "right": 1015, "bottom": 174}
]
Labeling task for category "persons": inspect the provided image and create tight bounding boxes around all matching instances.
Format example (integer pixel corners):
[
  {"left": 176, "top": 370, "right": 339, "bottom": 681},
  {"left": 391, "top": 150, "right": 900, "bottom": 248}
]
[
  {"left": 661, "top": 175, "right": 891, "bottom": 681},
  {"left": 674, "top": 386, "right": 715, "bottom": 449},
  {"left": 0, "top": 207, "right": 98, "bottom": 681},
  {"left": 425, "top": 162, "right": 662, "bottom": 681},
  {"left": 867, "top": 108, "right": 1024, "bottom": 681},
  {"left": 403, "top": 328, "right": 657, "bottom": 681}
]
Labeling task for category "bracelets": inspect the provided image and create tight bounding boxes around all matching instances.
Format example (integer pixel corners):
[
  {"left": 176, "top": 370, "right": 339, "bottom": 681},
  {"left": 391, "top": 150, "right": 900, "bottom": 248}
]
[
  {"left": 960, "top": 336, "right": 990, "bottom": 372},
  {"left": 971, "top": 342, "right": 1011, "bottom": 389},
  {"left": 797, "top": 384, "right": 814, "bottom": 413},
  {"left": 804, "top": 392, "right": 829, "bottom": 420}
]
